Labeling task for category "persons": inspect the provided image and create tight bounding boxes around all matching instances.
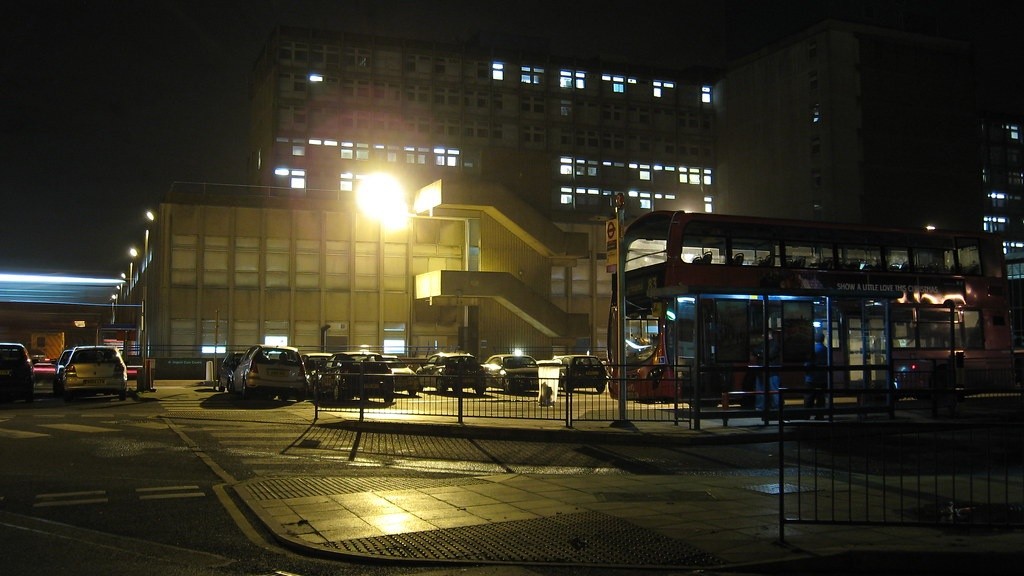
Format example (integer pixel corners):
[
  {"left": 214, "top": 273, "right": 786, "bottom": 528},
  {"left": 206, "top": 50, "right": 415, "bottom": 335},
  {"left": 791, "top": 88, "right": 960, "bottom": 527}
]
[{"left": 801, "top": 334, "right": 828, "bottom": 421}]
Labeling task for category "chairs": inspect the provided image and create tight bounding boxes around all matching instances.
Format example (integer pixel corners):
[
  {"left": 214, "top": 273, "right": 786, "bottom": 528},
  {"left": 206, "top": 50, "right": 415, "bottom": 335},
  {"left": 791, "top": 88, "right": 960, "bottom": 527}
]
[{"left": 693, "top": 253, "right": 980, "bottom": 275}]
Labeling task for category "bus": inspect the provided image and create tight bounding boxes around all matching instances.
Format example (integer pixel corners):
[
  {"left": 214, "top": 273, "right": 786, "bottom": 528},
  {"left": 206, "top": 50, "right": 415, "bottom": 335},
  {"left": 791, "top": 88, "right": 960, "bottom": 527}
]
[{"left": 606, "top": 211, "right": 1016, "bottom": 408}]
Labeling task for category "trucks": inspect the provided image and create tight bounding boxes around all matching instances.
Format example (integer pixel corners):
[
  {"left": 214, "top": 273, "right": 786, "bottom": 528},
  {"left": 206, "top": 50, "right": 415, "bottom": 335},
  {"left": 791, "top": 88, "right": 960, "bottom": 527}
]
[{"left": 16, "top": 331, "right": 64, "bottom": 360}]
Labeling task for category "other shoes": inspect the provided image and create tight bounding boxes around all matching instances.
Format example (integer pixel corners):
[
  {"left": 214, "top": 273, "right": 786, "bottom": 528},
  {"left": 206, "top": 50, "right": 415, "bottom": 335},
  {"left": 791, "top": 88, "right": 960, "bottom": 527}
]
[
  {"left": 932, "top": 413, "right": 938, "bottom": 419},
  {"left": 949, "top": 415, "right": 961, "bottom": 419}
]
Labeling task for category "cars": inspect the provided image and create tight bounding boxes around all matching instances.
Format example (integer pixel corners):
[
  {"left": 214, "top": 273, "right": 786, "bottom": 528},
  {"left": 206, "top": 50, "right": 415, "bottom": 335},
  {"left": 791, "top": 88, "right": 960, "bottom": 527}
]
[
  {"left": 32, "top": 359, "right": 57, "bottom": 378},
  {"left": 300, "top": 353, "right": 343, "bottom": 392},
  {"left": 29, "top": 350, "right": 46, "bottom": 361},
  {"left": 217, "top": 352, "right": 270, "bottom": 392},
  {"left": 0, "top": 343, "right": 36, "bottom": 404},
  {"left": 355, "top": 355, "right": 419, "bottom": 396},
  {"left": 50, "top": 345, "right": 128, "bottom": 401},
  {"left": 479, "top": 354, "right": 541, "bottom": 395},
  {"left": 314, "top": 351, "right": 395, "bottom": 403},
  {"left": 231, "top": 344, "right": 307, "bottom": 402},
  {"left": 552, "top": 355, "right": 607, "bottom": 393},
  {"left": 416, "top": 352, "right": 486, "bottom": 395}
]
